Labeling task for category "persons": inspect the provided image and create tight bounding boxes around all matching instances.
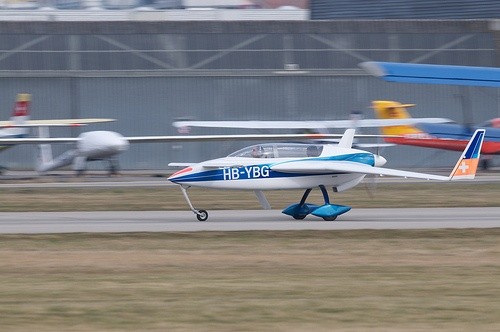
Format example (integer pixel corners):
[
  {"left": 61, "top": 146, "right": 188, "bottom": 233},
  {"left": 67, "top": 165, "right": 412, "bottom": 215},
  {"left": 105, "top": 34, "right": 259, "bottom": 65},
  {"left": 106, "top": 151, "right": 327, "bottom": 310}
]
[{"left": 252, "top": 146, "right": 267, "bottom": 158}]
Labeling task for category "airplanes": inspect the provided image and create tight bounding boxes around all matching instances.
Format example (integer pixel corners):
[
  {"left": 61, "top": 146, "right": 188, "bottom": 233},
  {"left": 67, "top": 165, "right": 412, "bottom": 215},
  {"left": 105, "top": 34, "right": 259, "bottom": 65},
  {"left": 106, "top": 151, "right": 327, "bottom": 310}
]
[{"left": 0, "top": 61, "right": 500, "bottom": 221}]
